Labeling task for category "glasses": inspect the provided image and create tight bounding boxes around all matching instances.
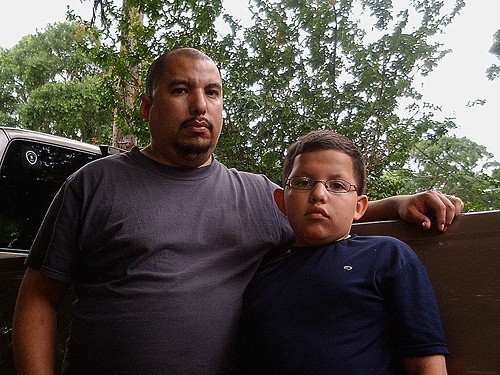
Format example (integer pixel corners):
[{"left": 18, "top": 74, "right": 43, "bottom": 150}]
[{"left": 284, "top": 175, "right": 360, "bottom": 194}]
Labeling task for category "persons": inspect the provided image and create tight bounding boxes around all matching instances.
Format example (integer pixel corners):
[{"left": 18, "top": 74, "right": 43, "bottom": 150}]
[
  {"left": 12, "top": 47, "right": 465, "bottom": 375},
  {"left": 236, "top": 129, "right": 450, "bottom": 375}
]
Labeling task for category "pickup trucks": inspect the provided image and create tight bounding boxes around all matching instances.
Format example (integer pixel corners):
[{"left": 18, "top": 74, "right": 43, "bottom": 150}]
[{"left": 0, "top": 127, "right": 500, "bottom": 375}]
